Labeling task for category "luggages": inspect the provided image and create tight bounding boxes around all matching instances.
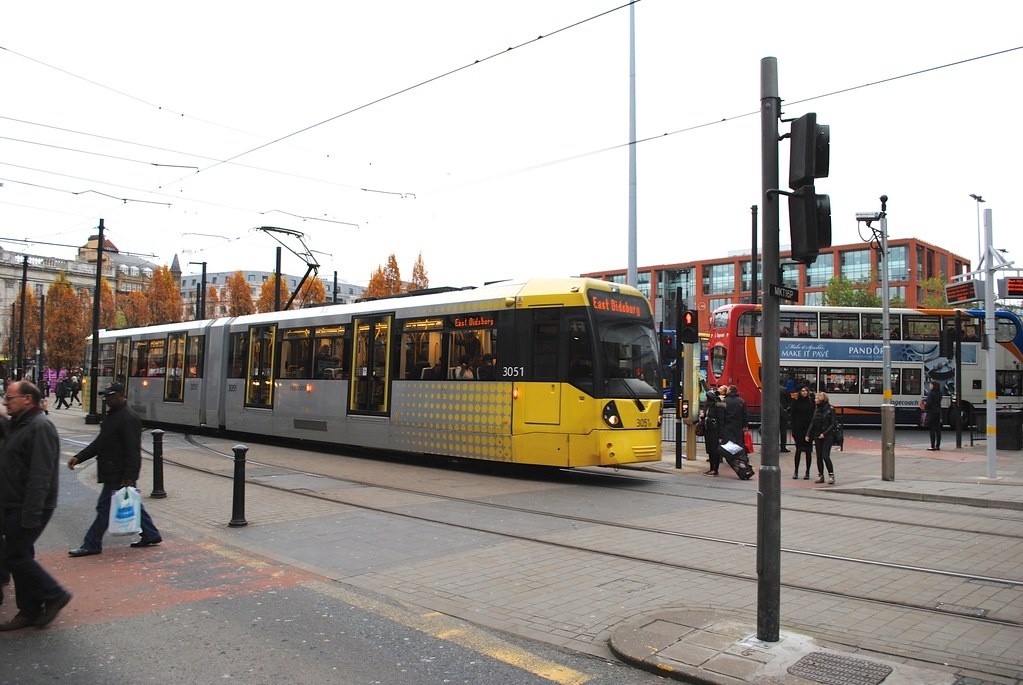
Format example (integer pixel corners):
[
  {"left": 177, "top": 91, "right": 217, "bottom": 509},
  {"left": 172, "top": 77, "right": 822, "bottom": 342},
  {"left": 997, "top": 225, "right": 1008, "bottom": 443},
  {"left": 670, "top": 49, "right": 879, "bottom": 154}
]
[{"left": 718, "top": 438, "right": 755, "bottom": 480}]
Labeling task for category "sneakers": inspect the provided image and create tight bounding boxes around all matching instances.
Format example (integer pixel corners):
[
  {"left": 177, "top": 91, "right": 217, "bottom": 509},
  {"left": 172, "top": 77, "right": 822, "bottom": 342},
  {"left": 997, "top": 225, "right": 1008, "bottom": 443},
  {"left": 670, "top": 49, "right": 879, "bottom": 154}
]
[
  {"left": 31, "top": 585, "right": 73, "bottom": 627},
  {"left": 0, "top": 612, "right": 37, "bottom": 631}
]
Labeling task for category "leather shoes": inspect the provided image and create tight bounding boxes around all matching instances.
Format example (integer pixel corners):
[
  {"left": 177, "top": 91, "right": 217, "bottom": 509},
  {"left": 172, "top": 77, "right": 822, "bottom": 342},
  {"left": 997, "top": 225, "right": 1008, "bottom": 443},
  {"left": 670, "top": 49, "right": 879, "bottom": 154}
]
[
  {"left": 130, "top": 536, "right": 162, "bottom": 548},
  {"left": 68, "top": 547, "right": 102, "bottom": 556}
]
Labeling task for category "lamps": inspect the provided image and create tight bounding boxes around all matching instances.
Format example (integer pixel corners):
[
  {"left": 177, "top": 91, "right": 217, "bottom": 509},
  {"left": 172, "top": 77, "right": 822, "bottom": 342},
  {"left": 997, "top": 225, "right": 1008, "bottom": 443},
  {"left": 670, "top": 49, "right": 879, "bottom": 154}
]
[{"left": 904, "top": 266, "right": 911, "bottom": 271}]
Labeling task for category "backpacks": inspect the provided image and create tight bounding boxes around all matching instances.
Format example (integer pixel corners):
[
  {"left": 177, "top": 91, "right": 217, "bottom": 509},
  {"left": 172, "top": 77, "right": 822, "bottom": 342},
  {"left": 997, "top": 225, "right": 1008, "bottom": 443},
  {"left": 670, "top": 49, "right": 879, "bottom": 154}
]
[{"left": 828, "top": 405, "right": 844, "bottom": 451}]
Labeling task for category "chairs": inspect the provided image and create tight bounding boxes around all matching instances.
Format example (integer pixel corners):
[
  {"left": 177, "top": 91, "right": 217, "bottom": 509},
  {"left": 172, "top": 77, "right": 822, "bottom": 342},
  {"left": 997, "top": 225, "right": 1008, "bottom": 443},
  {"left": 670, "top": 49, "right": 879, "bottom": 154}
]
[
  {"left": 420, "top": 366, "right": 494, "bottom": 380},
  {"left": 753, "top": 329, "right": 909, "bottom": 340},
  {"left": 321, "top": 368, "right": 342, "bottom": 378},
  {"left": 782, "top": 379, "right": 909, "bottom": 395}
]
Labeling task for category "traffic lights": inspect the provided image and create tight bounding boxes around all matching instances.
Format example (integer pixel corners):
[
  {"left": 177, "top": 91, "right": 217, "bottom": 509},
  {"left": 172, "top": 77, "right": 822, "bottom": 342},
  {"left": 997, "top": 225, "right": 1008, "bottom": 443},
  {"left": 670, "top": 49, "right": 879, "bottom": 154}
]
[
  {"left": 682, "top": 310, "right": 699, "bottom": 344},
  {"left": 665, "top": 338, "right": 671, "bottom": 357},
  {"left": 789, "top": 112, "right": 832, "bottom": 267}
]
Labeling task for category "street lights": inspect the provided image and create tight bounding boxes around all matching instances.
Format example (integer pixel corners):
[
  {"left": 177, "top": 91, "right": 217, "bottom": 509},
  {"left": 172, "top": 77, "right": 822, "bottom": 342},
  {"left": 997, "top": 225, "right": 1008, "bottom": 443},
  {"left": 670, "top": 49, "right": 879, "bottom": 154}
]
[{"left": 970, "top": 192, "right": 986, "bottom": 281}]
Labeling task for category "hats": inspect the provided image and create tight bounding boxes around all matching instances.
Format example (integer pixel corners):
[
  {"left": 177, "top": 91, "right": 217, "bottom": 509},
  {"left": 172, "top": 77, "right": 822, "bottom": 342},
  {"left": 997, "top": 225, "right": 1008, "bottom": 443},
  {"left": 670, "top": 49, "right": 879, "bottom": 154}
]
[
  {"left": 706, "top": 391, "right": 716, "bottom": 400},
  {"left": 797, "top": 383, "right": 807, "bottom": 390},
  {"left": 98, "top": 381, "right": 126, "bottom": 395}
]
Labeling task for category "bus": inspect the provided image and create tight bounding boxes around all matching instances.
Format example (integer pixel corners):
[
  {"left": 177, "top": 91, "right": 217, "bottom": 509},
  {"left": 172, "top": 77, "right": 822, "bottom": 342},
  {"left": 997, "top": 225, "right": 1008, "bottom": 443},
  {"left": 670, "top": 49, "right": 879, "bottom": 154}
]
[
  {"left": 80, "top": 276, "right": 666, "bottom": 469},
  {"left": 706, "top": 304, "right": 1023, "bottom": 432}
]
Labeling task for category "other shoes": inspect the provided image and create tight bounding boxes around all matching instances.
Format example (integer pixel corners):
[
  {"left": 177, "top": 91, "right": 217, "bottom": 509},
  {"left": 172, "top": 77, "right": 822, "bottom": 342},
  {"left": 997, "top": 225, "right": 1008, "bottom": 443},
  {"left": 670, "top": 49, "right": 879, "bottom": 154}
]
[
  {"left": 933, "top": 447, "right": 940, "bottom": 450},
  {"left": 828, "top": 475, "right": 836, "bottom": 484},
  {"left": 927, "top": 447, "right": 936, "bottom": 451},
  {"left": 803, "top": 471, "right": 810, "bottom": 480},
  {"left": 814, "top": 475, "right": 824, "bottom": 483},
  {"left": 793, "top": 472, "right": 799, "bottom": 479},
  {"left": 706, "top": 470, "right": 719, "bottom": 477},
  {"left": 703, "top": 470, "right": 712, "bottom": 476},
  {"left": 781, "top": 449, "right": 791, "bottom": 452}
]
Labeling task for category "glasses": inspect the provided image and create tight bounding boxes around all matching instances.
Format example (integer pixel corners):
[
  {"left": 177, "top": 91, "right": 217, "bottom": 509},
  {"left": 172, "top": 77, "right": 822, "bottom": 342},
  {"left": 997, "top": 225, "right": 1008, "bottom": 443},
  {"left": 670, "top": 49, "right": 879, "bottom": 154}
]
[{"left": 3, "top": 393, "right": 28, "bottom": 401}]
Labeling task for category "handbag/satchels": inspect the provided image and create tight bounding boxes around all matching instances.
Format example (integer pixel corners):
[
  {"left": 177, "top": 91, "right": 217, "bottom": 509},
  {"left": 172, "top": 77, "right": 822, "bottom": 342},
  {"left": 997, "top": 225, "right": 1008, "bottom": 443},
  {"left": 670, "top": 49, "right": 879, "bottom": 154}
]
[
  {"left": 743, "top": 430, "right": 754, "bottom": 454},
  {"left": 695, "top": 414, "right": 705, "bottom": 436},
  {"left": 108, "top": 486, "right": 143, "bottom": 536}
]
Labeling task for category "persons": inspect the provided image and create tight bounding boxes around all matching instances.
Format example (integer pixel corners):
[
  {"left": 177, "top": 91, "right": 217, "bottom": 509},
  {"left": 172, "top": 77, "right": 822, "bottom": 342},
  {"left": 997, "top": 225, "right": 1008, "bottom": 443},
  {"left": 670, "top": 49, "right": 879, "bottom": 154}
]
[
  {"left": 924, "top": 380, "right": 943, "bottom": 451},
  {"left": 67, "top": 380, "right": 162, "bottom": 557},
  {"left": 805, "top": 392, "right": 836, "bottom": 485},
  {"left": 698, "top": 384, "right": 750, "bottom": 478},
  {"left": 792, "top": 383, "right": 815, "bottom": 480},
  {"left": 779, "top": 377, "right": 791, "bottom": 453},
  {"left": 317, "top": 344, "right": 342, "bottom": 363},
  {"left": 2, "top": 374, "right": 82, "bottom": 410},
  {"left": 0, "top": 380, "right": 73, "bottom": 632},
  {"left": 455, "top": 331, "right": 495, "bottom": 379}
]
